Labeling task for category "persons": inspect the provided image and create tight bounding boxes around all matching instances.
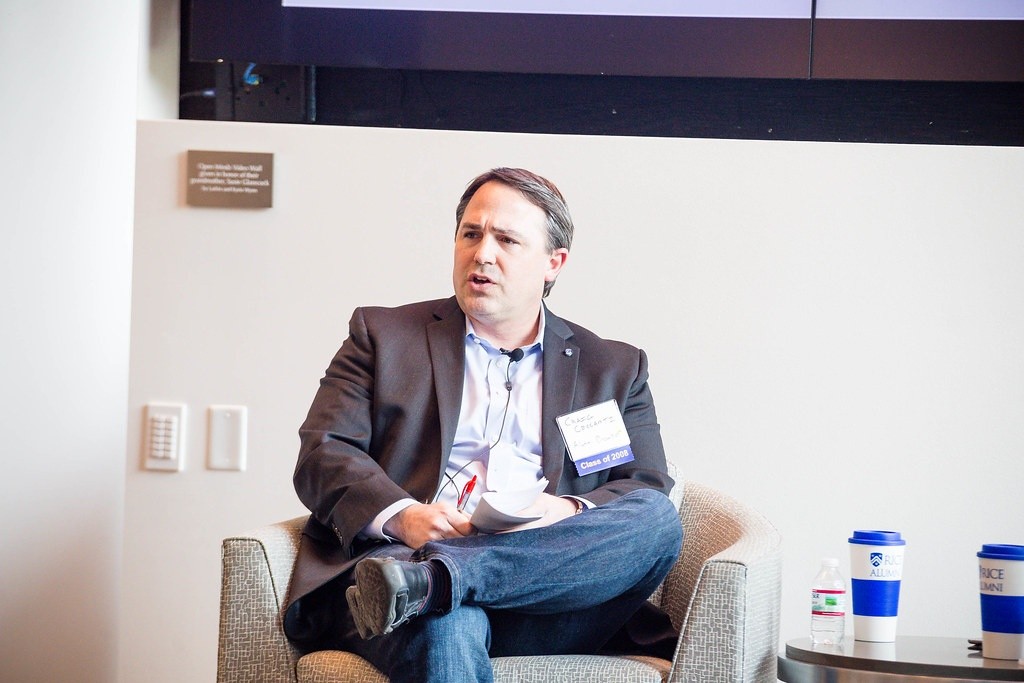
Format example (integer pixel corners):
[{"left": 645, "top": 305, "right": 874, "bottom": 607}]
[{"left": 284, "top": 167, "right": 684, "bottom": 683}]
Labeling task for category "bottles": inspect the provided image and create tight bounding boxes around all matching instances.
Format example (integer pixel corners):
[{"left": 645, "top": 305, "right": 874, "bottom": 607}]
[{"left": 810, "top": 558, "right": 846, "bottom": 644}]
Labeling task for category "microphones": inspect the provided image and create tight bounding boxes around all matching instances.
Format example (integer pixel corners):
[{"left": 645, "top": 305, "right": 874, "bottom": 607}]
[{"left": 510, "top": 349, "right": 524, "bottom": 362}]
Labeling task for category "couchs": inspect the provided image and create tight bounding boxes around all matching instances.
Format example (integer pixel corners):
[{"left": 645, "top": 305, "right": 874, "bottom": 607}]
[{"left": 216, "top": 478, "right": 780, "bottom": 683}]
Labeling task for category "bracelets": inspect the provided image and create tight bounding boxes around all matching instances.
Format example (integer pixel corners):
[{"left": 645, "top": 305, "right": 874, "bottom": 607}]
[{"left": 575, "top": 499, "right": 586, "bottom": 514}]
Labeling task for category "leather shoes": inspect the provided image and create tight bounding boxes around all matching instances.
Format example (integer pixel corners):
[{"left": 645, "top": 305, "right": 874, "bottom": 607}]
[{"left": 346, "top": 557, "right": 428, "bottom": 641}]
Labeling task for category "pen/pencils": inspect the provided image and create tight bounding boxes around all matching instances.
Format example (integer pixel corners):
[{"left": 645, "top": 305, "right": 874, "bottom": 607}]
[{"left": 456, "top": 475, "right": 477, "bottom": 513}]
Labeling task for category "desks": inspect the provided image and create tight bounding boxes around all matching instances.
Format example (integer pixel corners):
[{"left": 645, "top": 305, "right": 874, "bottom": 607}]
[{"left": 786, "top": 636, "right": 1024, "bottom": 683}]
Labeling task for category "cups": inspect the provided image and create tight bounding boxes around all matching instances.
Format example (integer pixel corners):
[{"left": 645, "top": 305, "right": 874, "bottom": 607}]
[
  {"left": 847, "top": 530, "right": 906, "bottom": 643},
  {"left": 977, "top": 543, "right": 1024, "bottom": 660}
]
